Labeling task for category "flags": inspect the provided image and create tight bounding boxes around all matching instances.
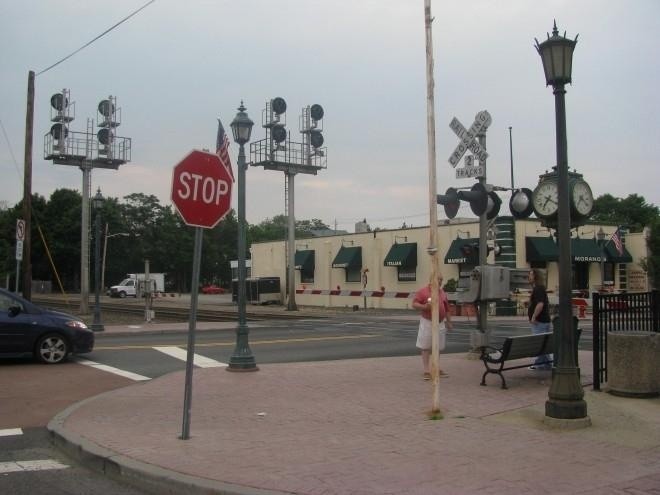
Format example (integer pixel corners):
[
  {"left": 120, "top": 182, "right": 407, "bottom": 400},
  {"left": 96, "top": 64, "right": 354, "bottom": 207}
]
[
  {"left": 612, "top": 228, "right": 625, "bottom": 258},
  {"left": 215, "top": 120, "right": 235, "bottom": 182}
]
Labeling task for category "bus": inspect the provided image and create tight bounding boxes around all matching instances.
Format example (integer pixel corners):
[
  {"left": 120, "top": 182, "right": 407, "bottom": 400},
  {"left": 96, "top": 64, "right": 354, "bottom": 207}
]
[{"left": 232, "top": 276, "right": 281, "bottom": 304}]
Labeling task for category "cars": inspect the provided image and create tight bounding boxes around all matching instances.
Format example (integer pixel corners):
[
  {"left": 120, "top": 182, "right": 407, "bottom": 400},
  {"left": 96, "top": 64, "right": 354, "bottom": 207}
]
[
  {"left": 203, "top": 284, "right": 225, "bottom": 294},
  {"left": 0, "top": 287, "right": 95, "bottom": 362}
]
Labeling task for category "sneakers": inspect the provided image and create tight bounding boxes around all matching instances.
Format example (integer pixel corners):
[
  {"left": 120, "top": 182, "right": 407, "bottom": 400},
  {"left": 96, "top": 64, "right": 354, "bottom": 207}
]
[
  {"left": 423, "top": 371, "right": 431, "bottom": 379},
  {"left": 439, "top": 369, "right": 448, "bottom": 378},
  {"left": 528, "top": 365, "right": 536, "bottom": 370}
]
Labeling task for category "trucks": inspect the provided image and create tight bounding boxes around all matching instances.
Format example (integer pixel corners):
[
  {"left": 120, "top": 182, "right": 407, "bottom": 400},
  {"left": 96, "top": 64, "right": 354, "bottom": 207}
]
[{"left": 111, "top": 273, "right": 165, "bottom": 298}]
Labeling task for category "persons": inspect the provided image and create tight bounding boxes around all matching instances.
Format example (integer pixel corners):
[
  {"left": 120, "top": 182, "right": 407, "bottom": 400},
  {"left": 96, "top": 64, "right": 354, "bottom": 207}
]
[
  {"left": 524, "top": 268, "right": 555, "bottom": 375},
  {"left": 411, "top": 269, "right": 451, "bottom": 382}
]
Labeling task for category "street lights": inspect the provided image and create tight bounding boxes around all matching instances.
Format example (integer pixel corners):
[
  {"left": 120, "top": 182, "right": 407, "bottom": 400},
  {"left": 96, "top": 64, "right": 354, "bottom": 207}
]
[
  {"left": 229, "top": 100, "right": 255, "bottom": 367},
  {"left": 533, "top": 19, "right": 589, "bottom": 418},
  {"left": 92, "top": 187, "right": 106, "bottom": 330},
  {"left": 101, "top": 223, "right": 141, "bottom": 293}
]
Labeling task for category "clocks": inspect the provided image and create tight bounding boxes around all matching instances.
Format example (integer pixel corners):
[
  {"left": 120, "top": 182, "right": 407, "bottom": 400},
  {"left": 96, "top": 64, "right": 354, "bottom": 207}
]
[{"left": 531, "top": 178, "right": 593, "bottom": 220}]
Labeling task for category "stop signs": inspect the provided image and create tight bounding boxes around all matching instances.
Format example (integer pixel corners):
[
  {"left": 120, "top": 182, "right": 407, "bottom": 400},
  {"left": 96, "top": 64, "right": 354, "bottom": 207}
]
[{"left": 171, "top": 151, "right": 233, "bottom": 231}]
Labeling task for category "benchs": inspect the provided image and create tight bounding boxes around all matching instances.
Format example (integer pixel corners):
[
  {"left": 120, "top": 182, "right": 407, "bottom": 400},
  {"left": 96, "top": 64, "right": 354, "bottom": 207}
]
[{"left": 475, "top": 328, "right": 582, "bottom": 390}]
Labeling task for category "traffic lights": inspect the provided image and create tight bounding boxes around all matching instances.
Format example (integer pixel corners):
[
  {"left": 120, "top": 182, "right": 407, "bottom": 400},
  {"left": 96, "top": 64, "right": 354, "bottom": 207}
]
[{"left": 460, "top": 244, "right": 475, "bottom": 264}]
